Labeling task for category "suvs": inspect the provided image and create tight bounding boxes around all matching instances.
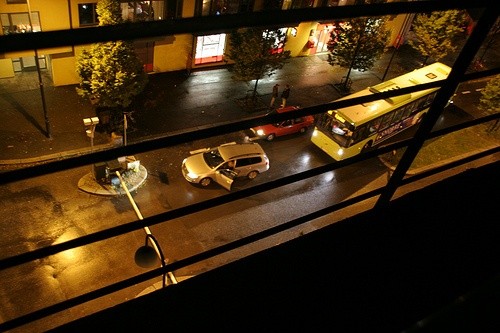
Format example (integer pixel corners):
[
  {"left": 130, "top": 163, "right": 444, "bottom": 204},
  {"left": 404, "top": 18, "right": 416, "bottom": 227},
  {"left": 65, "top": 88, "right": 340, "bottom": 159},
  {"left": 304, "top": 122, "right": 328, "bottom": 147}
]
[{"left": 181, "top": 142, "right": 271, "bottom": 188}]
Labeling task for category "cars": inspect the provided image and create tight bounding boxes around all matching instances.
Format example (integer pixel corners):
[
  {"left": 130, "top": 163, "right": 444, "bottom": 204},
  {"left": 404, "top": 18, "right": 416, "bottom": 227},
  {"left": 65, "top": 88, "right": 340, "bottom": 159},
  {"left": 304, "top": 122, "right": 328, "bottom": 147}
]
[{"left": 251, "top": 106, "right": 315, "bottom": 141}]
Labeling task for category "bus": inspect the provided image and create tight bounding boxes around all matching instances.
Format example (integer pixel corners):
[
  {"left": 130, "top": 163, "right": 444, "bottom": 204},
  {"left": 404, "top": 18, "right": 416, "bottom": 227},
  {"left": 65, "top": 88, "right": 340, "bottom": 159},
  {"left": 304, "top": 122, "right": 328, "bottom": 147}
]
[{"left": 311, "top": 61, "right": 458, "bottom": 161}]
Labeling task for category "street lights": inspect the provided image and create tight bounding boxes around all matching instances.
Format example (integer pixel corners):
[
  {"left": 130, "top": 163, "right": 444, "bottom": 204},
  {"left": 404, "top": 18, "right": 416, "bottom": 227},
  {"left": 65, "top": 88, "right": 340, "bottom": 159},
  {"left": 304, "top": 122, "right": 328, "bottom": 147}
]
[
  {"left": 83, "top": 117, "right": 99, "bottom": 153},
  {"left": 133, "top": 234, "right": 166, "bottom": 289}
]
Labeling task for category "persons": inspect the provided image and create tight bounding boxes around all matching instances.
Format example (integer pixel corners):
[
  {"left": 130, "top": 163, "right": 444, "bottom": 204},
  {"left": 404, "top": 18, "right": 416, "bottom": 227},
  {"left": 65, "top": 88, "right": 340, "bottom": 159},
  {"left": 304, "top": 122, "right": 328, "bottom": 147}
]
[
  {"left": 270, "top": 84, "right": 280, "bottom": 107},
  {"left": 279, "top": 84, "right": 290, "bottom": 109}
]
